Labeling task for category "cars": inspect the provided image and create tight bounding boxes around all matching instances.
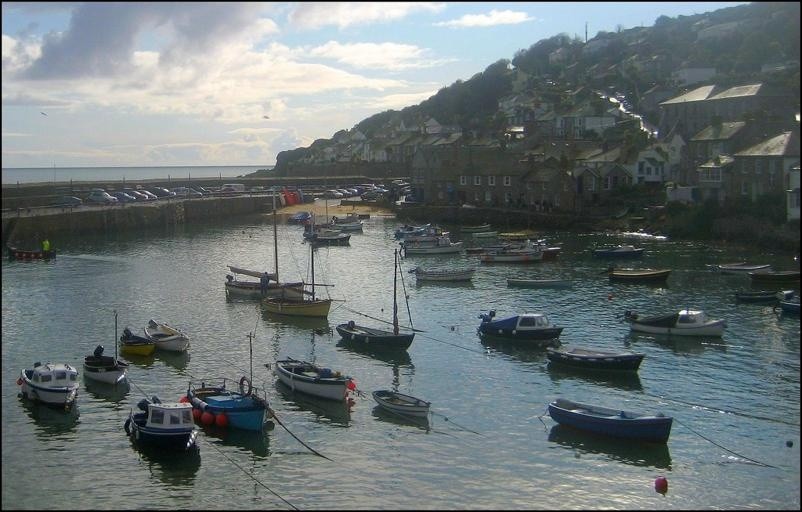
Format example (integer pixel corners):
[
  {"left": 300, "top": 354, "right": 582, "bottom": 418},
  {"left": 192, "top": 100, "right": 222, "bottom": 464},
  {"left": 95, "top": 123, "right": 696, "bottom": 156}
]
[
  {"left": 111, "top": 191, "right": 137, "bottom": 202},
  {"left": 128, "top": 178, "right": 411, "bottom": 204},
  {"left": 62, "top": 196, "right": 83, "bottom": 206}
]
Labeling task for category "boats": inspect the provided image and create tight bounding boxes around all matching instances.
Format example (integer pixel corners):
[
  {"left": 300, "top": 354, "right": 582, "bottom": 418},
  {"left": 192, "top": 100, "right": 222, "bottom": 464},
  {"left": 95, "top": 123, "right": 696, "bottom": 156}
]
[
  {"left": 409, "top": 264, "right": 475, "bottom": 281},
  {"left": 7, "top": 240, "right": 58, "bottom": 261},
  {"left": 777, "top": 288, "right": 800, "bottom": 314},
  {"left": 276, "top": 378, "right": 350, "bottom": 427},
  {"left": 373, "top": 390, "right": 432, "bottom": 418},
  {"left": 16, "top": 359, "right": 80, "bottom": 405},
  {"left": 129, "top": 398, "right": 196, "bottom": 446},
  {"left": 545, "top": 363, "right": 645, "bottom": 395},
  {"left": 83, "top": 311, "right": 131, "bottom": 384},
  {"left": 302, "top": 197, "right": 560, "bottom": 262},
  {"left": 414, "top": 277, "right": 476, "bottom": 292},
  {"left": 274, "top": 356, "right": 352, "bottom": 402},
  {"left": 478, "top": 333, "right": 562, "bottom": 369},
  {"left": 504, "top": 275, "right": 577, "bottom": 290},
  {"left": 622, "top": 306, "right": 727, "bottom": 339},
  {"left": 547, "top": 394, "right": 671, "bottom": 444},
  {"left": 117, "top": 326, "right": 155, "bottom": 356},
  {"left": 590, "top": 243, "right": 645, "bottom": 259},
  {"left": 155, "top": 350, "right": 190, "bottom": 371},
  {"left": 122, "top": 351, "right": 155, "bottom": 371},
  {"left": 126, "top": 434, "right": 201, "bottom": 484},
  {"left": 547, "top": 421, "right": 673, "bottom": 473},
  {"left": 202, "top": 425, "right": 272, "bottom": 465},
  {"left": 716, "top": 264, "right": 773, "bottom": 274},
  {"left": 83, "top": 378, "right": 130, "bottom": 410},
  {"left": 605, "top": 264, "right": 673, "bottom": 280},
  {"left": 285, "top": 211, "right": 312, "bottom": 225},
  {"left": 625, "top": 330, "right": 728, "bottom": 359},
  {"left": 734, "top": 286, "right": 794, "bottom": 302},
  {"left": 371, "top": 405, "right": 431, "bottom": 435},
  {"left": 18, "top": 395, "right": 82, "bottom": 441},
  {"left": 541, "top": 341, "right": 645, "bottom": 372},
  {"left": 477, "top": 310, "right": 566, "bottom": 340},
  {"left": 180, "top": 333, "right": 269, "bottom": 433},
  {"left": 747, "top": 269, "right": 800, "bottom": 281},
  {"left": 144, "top": 319, "right": 189, "bottom": 353}
]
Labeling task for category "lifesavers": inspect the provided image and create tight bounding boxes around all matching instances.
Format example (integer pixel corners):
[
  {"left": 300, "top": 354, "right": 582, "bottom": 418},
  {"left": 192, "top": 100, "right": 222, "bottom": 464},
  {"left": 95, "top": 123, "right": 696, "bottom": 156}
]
[{"left": 240, "top": 377, "right": 252, "bottom": 396}]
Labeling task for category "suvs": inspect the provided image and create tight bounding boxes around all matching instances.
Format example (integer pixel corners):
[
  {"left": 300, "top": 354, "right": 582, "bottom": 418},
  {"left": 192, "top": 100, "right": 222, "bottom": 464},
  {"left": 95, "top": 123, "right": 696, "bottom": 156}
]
[{"left": 87, "top": 187, "right": 118, "bottom": 204}]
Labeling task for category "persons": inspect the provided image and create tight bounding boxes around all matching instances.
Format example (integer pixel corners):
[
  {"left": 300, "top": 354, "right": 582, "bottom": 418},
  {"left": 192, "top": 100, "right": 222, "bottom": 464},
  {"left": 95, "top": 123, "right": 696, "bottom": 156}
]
[{"left": 41, "top": 235, "right": 50, "bottom": 259}]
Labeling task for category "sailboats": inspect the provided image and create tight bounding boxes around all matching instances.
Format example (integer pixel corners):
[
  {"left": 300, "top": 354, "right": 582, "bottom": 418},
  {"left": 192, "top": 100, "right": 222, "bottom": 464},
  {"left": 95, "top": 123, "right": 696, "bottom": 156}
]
[
  {"left": 261, "top": 309, "right": 332, "bottom": 364},
  {"left": 336, "top": 246, "right": 417, "bottom": 351},
  {"left": 337, "top": 338, "right": 415, "bottom": 390},
  {"left": 259, "top": 208, "right": 334, "bottom": 319},
  {"left": 223, "top": 185, "right": 307, "bottom": 299}
]
